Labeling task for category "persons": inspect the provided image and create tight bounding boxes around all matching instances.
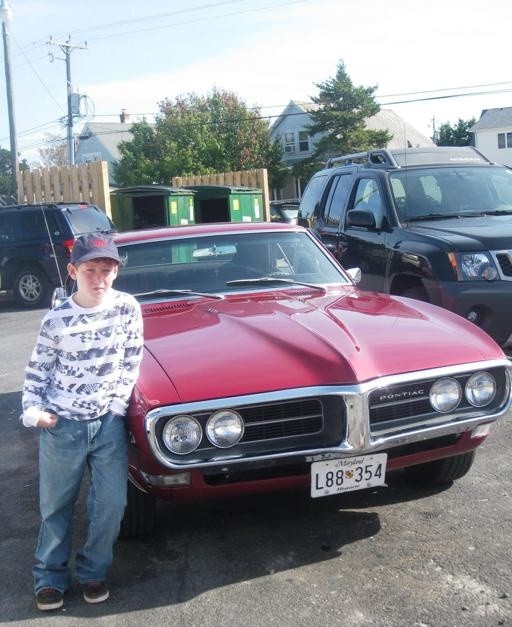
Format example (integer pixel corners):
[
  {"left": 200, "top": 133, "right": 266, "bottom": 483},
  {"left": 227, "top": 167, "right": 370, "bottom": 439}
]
[{"left": 21, "top": 232, "right": 143, "bottom": 611}]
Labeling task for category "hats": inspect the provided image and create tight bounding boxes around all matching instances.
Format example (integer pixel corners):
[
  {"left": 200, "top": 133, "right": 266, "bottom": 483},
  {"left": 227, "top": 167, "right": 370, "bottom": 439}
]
[{"left": 71, "top": 234, "right": 121, "bottom": 265}]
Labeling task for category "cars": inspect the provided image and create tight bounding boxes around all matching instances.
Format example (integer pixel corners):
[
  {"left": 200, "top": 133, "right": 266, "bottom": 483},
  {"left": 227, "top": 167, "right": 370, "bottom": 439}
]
[{"left": 84, "top": 223, "right": 511, "bottom": 511}]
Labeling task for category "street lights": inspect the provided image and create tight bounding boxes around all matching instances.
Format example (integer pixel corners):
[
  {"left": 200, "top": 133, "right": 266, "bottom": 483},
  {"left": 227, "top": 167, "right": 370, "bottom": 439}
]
[{"left": 1, "top": 3, "right": 19, "bottom": 201}]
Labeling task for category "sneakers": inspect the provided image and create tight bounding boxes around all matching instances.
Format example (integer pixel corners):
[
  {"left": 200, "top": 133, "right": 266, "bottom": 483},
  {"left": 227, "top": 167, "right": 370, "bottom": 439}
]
[
  {"left": 81, "top": 581, "right": 109, "bottom": 604},
  {"left": 36, "top": 588, "right": 63, "bottom": 610}
]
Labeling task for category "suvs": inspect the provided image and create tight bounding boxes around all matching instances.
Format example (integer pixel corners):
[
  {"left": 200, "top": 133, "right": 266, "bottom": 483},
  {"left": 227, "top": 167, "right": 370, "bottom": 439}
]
[
  {"left": 0, "top": 201, "right": 119, "bottom": 308},
  {"left": 298, "top": 146, "right": 511, "bottom": 348}
]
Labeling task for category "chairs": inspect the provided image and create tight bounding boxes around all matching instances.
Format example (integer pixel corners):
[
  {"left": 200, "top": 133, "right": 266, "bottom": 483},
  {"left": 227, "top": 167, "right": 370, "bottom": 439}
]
[{"left": 365, "top": 193, "right": 403, "bottom": 226}]
